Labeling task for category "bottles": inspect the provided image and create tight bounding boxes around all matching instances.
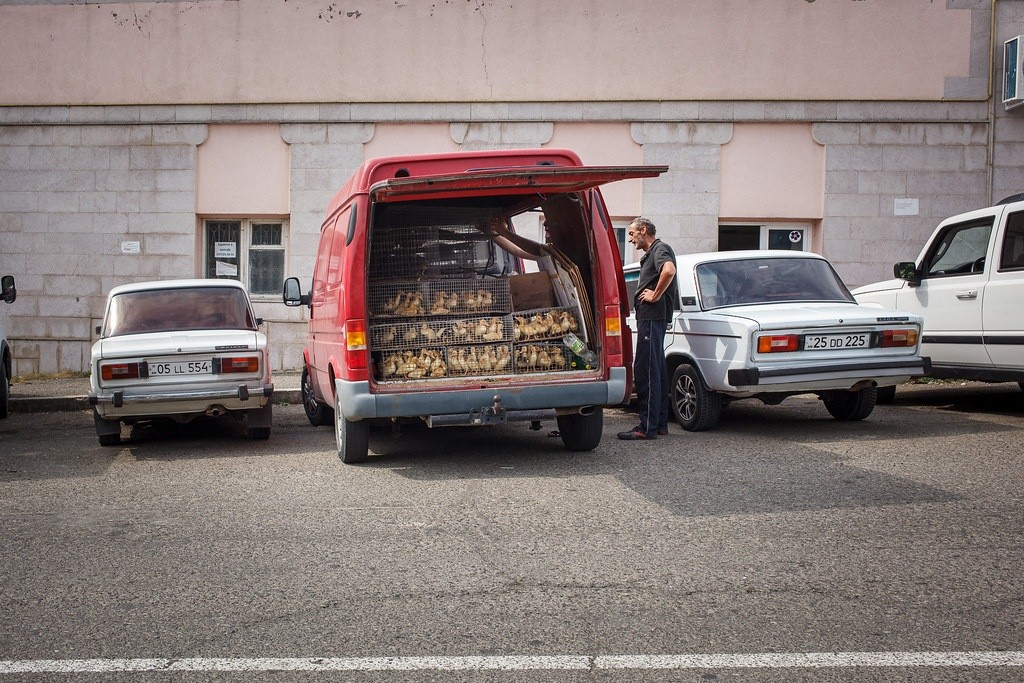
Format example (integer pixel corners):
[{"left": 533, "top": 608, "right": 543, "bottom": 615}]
[{"left": 563, "top": 332, "right": 599, "bottom": 368}]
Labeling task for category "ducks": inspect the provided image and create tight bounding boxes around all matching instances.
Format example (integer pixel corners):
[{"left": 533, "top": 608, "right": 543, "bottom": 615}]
[{"left": 379, "top": 290, "right": 578, "bottom": 379}]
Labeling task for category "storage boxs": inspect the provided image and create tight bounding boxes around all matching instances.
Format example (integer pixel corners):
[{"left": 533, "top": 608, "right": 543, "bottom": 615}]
[{"left": 369, "top": 216, "right": 586, "bottom": 380}]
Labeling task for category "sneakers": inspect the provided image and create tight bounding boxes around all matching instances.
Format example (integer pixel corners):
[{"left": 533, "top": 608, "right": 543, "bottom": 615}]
[{"left": 616, "top": 426, "right": 668, "bottom": 439}]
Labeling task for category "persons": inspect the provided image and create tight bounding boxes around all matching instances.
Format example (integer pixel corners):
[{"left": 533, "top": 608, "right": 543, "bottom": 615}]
[
  {"left": 617, "top": 218, "right": 677, "bottom": 439},
  {"left": 476, "top": 216, "right": 560, "bottom": 437}
]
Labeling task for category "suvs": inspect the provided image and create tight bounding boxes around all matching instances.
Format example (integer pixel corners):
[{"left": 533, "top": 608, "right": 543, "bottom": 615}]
[{"left": 848, "top": 200, "right": 1024, "bottom": 405}]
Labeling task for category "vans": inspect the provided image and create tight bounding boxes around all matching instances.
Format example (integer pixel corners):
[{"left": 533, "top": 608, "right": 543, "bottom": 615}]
[{"left": 280, "top": 147, "right": 674, "bottom": 466}]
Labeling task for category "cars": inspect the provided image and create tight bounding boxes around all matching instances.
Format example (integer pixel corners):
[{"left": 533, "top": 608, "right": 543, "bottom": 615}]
[
  {"left": 88, "top": 278, "right": 275, "bottom": 448},
  {"left": 0, "top": 276, "right": 17, "bottom": 419},
  {"left": 617, "top": 249, "right": 933, "bottom": 435}
]
